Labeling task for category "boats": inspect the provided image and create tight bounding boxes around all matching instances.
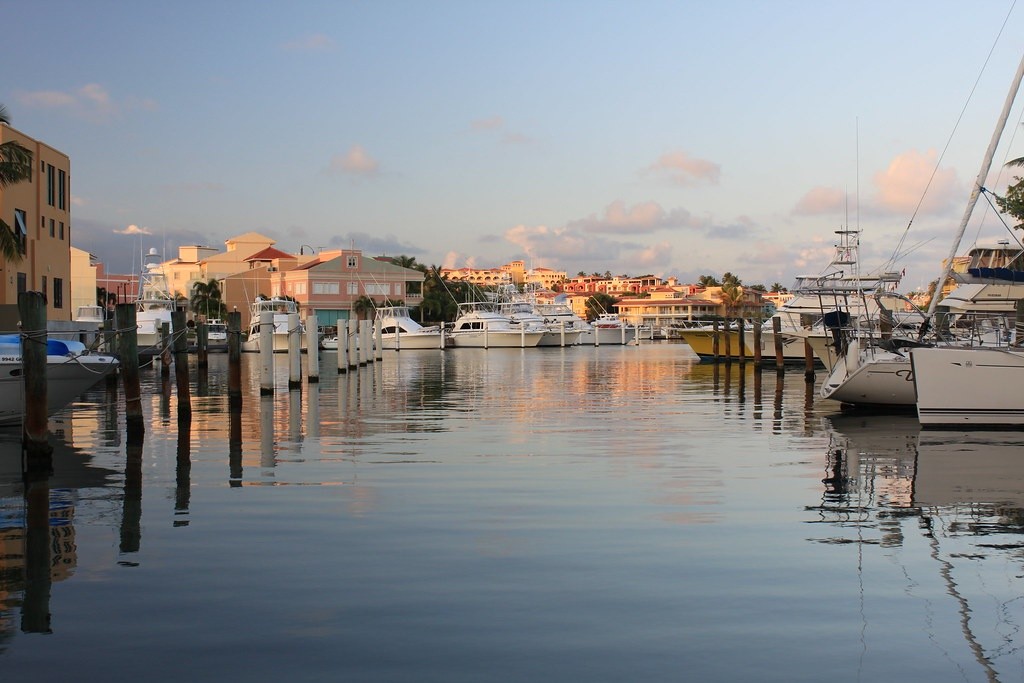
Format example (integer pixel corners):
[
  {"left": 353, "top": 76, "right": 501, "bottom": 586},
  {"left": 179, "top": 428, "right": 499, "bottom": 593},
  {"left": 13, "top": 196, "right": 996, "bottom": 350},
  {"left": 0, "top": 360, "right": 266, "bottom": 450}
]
[
  {"left": 118, "top": 230, "right": 175, "bottom": 358},
  {"left": 319, "top": 268, "right": 443, "bottom": 350},
  {"left": 0, "top": 334, "right": 120, "bottom": 433},
  {"left": 427, "top": 2, "right": 1024, "bottom": 429},
  {"left": 240, "top": 265, "right": 328, "bottom": 354},
  {"left": 184, "top": 291, "right": 230, "bottom": 356}
]
